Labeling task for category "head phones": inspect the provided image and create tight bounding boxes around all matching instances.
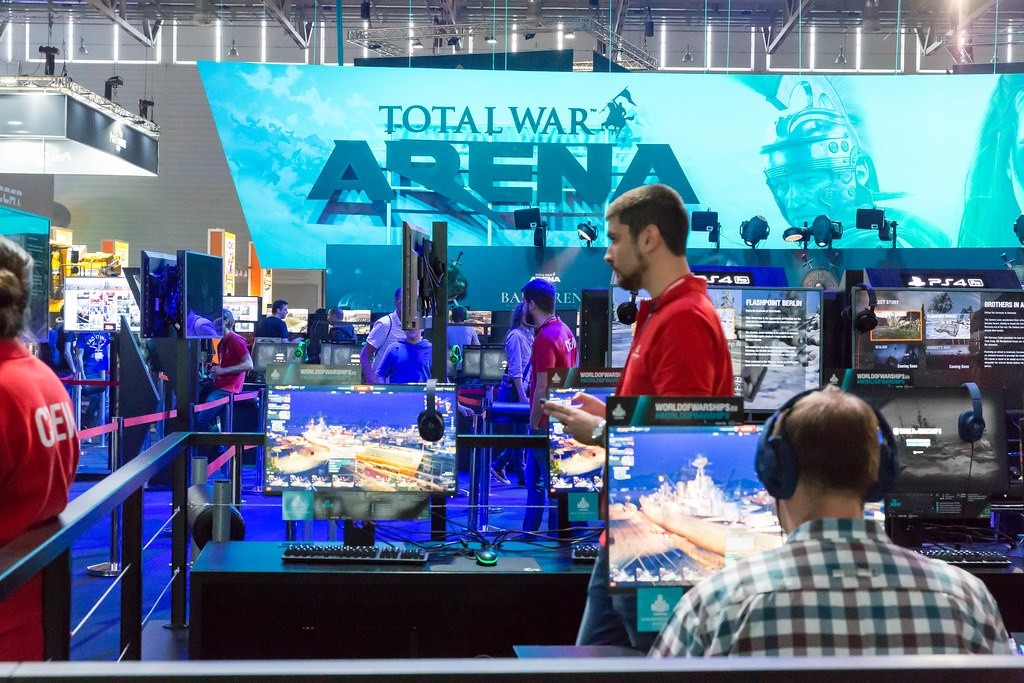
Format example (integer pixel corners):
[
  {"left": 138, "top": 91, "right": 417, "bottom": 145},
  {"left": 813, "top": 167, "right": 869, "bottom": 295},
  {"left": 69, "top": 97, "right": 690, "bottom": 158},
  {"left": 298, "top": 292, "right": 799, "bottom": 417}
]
[
  {"left": 294, "top": 341, "right": 309, "bottom": 362},
  {"left": 450, "top": 344, "right": 462, "bottom": 365},
  {"left": 754, "top": 387, "right": 899, "bottom": 502},
  {"left": 449, "top": 306, "right": 470, "bottom": 320},
  {"left": 617, "top": 293, "right": 639, "bottom": 326},
  {"left": 958, "top": 383, "right": 985, "bottom": 443},
  {"left": 841, "top": 283, "right": 878, "bottom": 333},
  {"left": 417, "top": 379, "right": 445, "bottom": 442}
]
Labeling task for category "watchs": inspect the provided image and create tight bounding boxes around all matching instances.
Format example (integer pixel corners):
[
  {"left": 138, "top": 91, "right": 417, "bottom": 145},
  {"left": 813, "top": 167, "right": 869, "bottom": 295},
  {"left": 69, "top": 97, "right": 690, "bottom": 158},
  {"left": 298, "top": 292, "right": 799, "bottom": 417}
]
[{"left": 592, "top": 419, "right": 607, "bottom": 448}]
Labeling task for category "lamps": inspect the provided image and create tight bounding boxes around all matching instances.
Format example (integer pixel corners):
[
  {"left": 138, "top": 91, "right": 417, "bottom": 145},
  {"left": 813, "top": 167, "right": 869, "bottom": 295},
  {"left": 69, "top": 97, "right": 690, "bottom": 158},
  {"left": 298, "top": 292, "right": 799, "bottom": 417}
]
[
  {"left": 577, "top": 221, "right": 598, "bottom": 247},
  {"left": 783, "top": 222, "right": 809, "bottom": 248},
  {"left": 739, "top": 214, "right": 770, "bottom": 250},
  {"left": 812, "top": 215, "right": 843, "bottom": 249}
]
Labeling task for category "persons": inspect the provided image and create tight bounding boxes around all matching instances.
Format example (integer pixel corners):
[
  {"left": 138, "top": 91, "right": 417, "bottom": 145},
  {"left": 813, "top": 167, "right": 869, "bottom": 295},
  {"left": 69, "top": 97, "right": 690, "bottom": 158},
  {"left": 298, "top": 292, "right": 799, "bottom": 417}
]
[
  {"left": 186, "top": 301, "right": 219, "bottom": 335},
  {"left": 646, "top": 383, "right": 1014, "bottom": 658},
  {"left": 196, "top": 309, "right": 254, "bottom": 474},
  {"left": 804, "top": 269, "right": 839, "bottom": 290},
  {"left": 541, "top": 183, "right": 734, "bottom": 651},
  {"left": 0, "top": 234, "right": 81, "bottom": 662},
  {"left": 53, "top": 305, "right": 76, "bottom": 397},
  {"left": 75, "top": 330, "right": 111, "bottom": 443},
  {"left": 255, "top": 277, "right": 578, "bottom": 541}
]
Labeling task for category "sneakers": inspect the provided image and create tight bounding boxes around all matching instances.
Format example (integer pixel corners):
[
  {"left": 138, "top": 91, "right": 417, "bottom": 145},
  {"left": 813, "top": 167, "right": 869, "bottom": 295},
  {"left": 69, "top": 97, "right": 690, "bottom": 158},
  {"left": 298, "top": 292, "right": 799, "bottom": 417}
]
[
  {"left": 519, "top": 478, "right": 526, "bottom": 488},
  {"left": 491, "top": 464, "right": 511, "bottom": 485}
]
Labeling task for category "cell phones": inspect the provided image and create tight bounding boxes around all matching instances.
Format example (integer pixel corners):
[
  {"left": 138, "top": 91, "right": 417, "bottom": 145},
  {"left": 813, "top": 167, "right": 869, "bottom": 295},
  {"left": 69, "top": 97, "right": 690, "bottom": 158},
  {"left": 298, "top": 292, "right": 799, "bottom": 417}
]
[{"left": 539, "top": 397, "right": 548, "bottom": 404}]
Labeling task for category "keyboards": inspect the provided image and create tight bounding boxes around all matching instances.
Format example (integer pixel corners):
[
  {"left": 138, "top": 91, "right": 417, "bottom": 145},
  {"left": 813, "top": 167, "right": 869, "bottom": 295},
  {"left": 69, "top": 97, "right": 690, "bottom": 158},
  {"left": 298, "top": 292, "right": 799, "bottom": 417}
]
[
  {"left": 911, "top": 549, "right": 1012, "bottom": 567},
  {"left": 570, "top": 544, "right": 600, "bottom": 562},
  {"left": 282, "top": 545, "right": 428, "bottom": 564}
]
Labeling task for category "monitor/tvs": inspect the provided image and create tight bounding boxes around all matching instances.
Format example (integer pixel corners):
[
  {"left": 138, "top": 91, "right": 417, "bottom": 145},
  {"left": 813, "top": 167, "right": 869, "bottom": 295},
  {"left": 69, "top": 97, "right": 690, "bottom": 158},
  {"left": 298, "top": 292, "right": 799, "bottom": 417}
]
[{"left": 0, "top": 206, "right": 1024, "bottom": 593}]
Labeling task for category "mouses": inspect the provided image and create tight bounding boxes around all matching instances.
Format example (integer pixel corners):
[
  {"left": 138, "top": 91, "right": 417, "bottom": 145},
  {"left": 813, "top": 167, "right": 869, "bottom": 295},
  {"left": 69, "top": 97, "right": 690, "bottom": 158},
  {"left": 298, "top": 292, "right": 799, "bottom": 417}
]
[{"left": 476, "top": 551, "right": 499, "bottom": 566}]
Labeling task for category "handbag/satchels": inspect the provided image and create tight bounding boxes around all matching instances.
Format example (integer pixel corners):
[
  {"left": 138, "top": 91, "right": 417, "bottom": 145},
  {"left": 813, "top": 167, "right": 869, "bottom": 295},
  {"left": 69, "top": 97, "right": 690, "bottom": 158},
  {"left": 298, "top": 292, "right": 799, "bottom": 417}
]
[{"left": 491, "top": 375, "right": 530, "bottom": 424}]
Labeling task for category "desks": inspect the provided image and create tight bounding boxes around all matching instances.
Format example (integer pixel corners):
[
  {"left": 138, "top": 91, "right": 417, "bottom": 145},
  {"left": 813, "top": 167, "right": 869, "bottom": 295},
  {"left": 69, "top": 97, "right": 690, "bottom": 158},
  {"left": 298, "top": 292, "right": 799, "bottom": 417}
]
[{"left": 188, "top": 540, "right": 1024, "bottom": 659}]
